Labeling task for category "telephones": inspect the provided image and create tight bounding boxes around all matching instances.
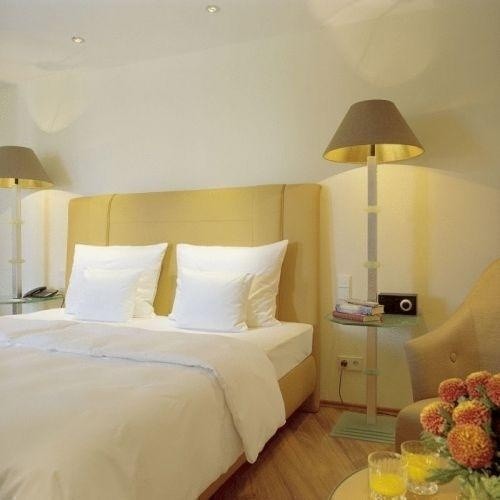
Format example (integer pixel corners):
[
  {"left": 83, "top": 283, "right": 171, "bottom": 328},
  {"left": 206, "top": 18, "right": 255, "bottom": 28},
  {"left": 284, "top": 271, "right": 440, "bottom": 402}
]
[{"left": 23, "top": 286, "right": 58, "bottom": 299}]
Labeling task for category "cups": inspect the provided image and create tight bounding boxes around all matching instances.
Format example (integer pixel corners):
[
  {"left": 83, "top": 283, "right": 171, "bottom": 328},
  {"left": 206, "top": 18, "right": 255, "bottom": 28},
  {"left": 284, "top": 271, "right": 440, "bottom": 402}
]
[
  {"left": 402, "top": 440, "right": 442, "bottom": 495},
  {"left": 368, "top": 451, "right": 409, "bottom": 500}
]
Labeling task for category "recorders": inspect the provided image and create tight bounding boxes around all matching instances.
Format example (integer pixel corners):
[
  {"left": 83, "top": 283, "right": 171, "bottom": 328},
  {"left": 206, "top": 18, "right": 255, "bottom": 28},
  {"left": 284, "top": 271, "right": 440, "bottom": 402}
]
[{"left": 377, "top": 292, "right": 417, "bottom": 315}]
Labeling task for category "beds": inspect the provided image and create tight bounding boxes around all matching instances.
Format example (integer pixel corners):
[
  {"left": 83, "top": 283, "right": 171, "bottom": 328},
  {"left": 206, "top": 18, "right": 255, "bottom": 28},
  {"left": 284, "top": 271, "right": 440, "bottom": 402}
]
[{"left": 0, "top": 183, "right": 324, "bottom": 500}]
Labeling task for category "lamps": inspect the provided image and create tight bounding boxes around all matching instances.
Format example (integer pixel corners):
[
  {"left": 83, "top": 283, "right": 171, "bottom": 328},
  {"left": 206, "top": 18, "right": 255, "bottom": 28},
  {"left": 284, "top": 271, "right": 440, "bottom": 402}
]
[
  {"left": 0, "top": 145, "right": 55, "bottom": 316},
  {"left": 322, "top": 98, "right": 425, "bottom": 444}
]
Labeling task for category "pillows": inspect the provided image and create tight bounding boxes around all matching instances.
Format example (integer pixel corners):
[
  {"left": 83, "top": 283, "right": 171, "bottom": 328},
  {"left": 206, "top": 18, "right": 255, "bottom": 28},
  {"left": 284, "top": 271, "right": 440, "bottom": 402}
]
[
  {"left": 169, "top": 266, "right": 255, "bottom": 333},
  {"left": 168, "top": 238, "right": 289, "bottom": 328},
  {"left": 72, "top": 268, "right": 142, "bottom": 323},
  {"left": 65, "top": 242, "right": 168, "bottom": 318}
]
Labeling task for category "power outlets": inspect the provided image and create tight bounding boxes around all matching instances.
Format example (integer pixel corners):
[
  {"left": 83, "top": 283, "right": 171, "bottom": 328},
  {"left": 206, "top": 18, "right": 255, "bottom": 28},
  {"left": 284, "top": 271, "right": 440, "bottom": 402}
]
[{"left": 340, "top": 357, "right": 364, "bottom": 372}]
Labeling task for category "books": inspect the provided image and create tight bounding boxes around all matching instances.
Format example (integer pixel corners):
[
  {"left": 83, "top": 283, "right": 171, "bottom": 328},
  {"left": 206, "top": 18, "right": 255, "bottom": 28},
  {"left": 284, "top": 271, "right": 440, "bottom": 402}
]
[
  {"left": 332, "top": 315, "right": 385, "bottom": 322},
  {"left": 348, "top": 299, "right": 378, "bottom": 307},
  {"left": 335, "top": 304, "right": 384, "bottom": 315}
]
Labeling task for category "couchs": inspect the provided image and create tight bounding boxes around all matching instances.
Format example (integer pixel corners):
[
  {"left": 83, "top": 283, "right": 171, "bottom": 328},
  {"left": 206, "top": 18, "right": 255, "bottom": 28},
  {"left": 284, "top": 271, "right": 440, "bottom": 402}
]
[{"left": 395, "top": 258, "right": 500, "bottom": 454}]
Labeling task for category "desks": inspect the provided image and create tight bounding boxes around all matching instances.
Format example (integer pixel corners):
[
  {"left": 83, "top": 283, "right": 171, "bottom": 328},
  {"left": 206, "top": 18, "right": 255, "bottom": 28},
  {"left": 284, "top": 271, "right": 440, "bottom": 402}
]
[{"left": 0, "top": 295, "right": 64, "bottom": 315}]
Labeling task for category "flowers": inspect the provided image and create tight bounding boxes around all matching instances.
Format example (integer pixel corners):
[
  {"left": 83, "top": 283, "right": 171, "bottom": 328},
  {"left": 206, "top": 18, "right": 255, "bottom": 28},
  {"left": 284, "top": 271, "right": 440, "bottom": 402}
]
[{"left": 419, "top": 371, "right": 500, "bottom": 470}]
